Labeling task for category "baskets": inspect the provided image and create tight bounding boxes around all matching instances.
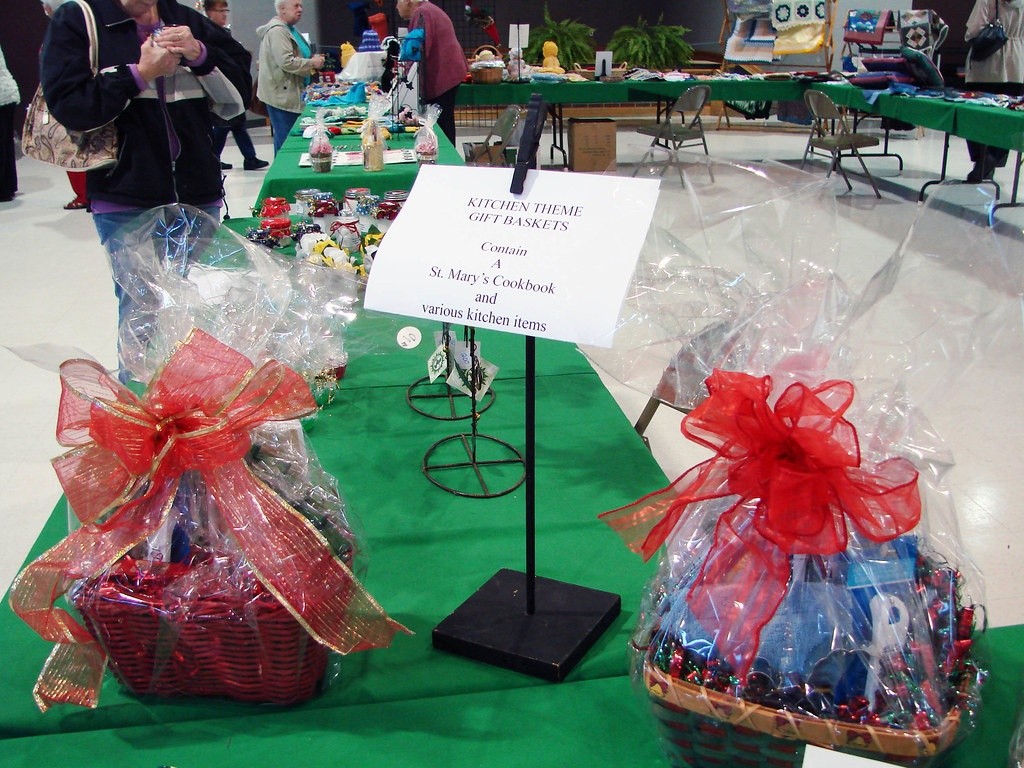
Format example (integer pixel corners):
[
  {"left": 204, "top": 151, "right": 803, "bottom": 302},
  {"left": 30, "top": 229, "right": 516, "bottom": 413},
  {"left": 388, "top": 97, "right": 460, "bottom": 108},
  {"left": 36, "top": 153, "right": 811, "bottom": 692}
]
[
  {"left": 645, "top": 641, "right": 968, "bottom": 768},
  {"left": 470, "top": 45, "right": 504, "bottom": 84},
  {"left": 73, "top": 552, "right": 355, "bottom": 706}
]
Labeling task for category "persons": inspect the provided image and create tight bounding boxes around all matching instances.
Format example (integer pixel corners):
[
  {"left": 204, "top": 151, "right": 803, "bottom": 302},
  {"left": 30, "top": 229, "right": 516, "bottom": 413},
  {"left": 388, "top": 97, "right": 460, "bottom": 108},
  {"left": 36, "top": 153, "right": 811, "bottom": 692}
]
[
  {"left": 63, "top": 172, "right": 87, "bottom": 210},
  {"left": 0, "top": 49, "right": 21, "bottom": 204},
  {"left": 200, "top": 0, "right": 269, "bottom": 171},
  {"left": 256, "top": 0, "right": 325, "bottom": 160},
  {"left": 964, "top": 0, "right": 1024, "bottom": 183},
  {"left": 39, "top": 0, "right": 252, "bottom": 384},
  {"left": 396, "top": 0, "right": 468, "bottom": 149}
]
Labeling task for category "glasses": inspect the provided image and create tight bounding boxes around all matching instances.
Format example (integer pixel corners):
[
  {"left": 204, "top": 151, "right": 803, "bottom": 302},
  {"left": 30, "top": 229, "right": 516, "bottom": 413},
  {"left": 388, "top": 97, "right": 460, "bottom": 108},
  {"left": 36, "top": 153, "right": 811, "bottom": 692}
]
[{"left": 209, "top": 8, "right": 230, "bottom": 14}]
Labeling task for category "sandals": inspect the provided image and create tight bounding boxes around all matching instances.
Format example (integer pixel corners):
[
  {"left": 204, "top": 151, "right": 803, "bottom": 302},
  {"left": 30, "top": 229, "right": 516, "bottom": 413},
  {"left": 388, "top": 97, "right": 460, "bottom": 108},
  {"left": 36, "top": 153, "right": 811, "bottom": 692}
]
[{"left": 63, "top": 199, "right": 86, "bottom": 209}]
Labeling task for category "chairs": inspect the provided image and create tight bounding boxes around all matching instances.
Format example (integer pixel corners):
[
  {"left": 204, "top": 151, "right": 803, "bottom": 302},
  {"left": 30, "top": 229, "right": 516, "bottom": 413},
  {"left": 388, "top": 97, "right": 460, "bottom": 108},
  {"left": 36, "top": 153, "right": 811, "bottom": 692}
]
[
  {"left": 466, "top": 103, "right": 521, "bottom": 170},
  {"left": 633, "top": 85, "right": 714, "bottom": 189},
  {"left": 800, "top": 91, "right": 880, "bottom": 198}
]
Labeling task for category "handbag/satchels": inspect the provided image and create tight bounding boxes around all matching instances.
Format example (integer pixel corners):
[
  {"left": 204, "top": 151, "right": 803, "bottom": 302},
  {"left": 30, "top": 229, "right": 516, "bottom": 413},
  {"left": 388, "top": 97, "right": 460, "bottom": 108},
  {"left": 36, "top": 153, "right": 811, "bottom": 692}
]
[
  {"left": 21, "top": 0, "right": 120, "bottom": 174},
  {"left": 970, "top": 0, "right": 1009, "bottom": 62},
  {"left": 249, "top": 77, "right": 270, "bottom": 117}
]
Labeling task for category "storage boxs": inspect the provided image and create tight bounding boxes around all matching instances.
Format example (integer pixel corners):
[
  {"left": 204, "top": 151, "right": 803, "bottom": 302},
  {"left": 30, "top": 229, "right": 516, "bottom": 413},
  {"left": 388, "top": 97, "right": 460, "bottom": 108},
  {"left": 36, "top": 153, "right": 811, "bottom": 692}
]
[
  {"left": 569, "top": 118, "right": 617, "bottom": 174},
  {"left": 461, "top": 142, "right": 508, "bottom": 167}
]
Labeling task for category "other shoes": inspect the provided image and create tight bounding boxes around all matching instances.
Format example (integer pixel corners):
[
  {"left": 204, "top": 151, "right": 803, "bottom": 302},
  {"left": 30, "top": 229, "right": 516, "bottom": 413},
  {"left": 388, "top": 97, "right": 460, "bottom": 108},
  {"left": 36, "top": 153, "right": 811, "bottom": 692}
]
[
  {"left": 967, "top": 164, "right": 983, "bottom": 184},
  {"left": 244, "top": 157, "right": 269, "bottom": 170},
  {"left": 221, "top": 162, "right": 233, "bottom": 170},
  {"left": 984, "top": 167, "right": 996, "bottom": 179}
]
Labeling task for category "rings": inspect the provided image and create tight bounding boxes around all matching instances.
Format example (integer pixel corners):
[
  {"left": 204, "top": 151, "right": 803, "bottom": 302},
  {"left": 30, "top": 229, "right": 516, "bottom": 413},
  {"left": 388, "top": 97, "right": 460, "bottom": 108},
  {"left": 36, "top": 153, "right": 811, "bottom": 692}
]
[{"left": 177, "top": 34, "right": 180, "bottom": 40}]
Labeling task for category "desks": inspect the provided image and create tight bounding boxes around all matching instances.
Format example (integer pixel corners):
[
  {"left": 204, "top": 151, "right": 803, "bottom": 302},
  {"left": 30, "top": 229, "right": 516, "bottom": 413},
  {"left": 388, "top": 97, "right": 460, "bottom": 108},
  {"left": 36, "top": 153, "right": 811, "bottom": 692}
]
[
  {"left": 254, "top": 73, "right": 810, "bottom": 207},
  {"left": 811, "top": 69, "right": 1024, "bottom": 227},
  {"left": 0, "top": 216, "right": 1024, "bottom": 768}
]
